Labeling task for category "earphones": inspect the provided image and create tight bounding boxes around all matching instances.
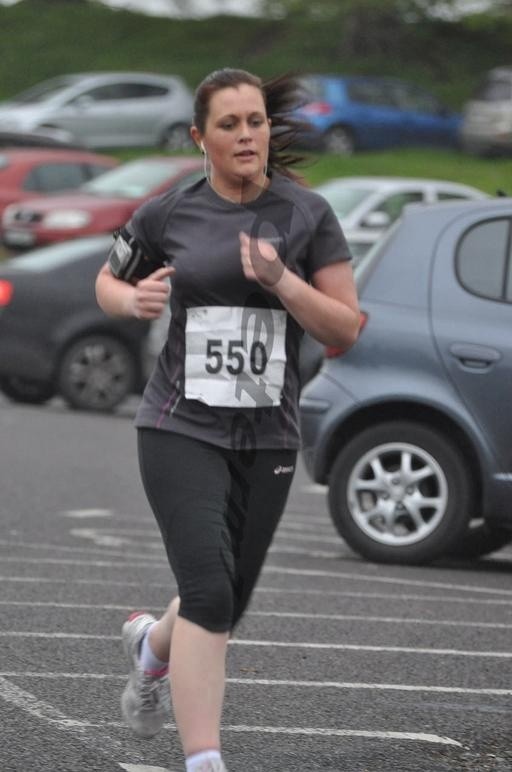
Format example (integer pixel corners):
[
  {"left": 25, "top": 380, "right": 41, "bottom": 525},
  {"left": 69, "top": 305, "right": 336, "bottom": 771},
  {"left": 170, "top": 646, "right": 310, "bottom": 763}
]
[{"left": 200, "top": 140, "right": 207, "bottom": 154}]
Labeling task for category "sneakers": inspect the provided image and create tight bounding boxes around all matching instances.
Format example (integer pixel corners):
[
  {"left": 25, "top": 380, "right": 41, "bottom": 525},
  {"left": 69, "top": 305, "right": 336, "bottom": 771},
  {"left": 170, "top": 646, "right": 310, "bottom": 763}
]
[{"left": 115, "top": 608, "right": 175, "bottom": 741}]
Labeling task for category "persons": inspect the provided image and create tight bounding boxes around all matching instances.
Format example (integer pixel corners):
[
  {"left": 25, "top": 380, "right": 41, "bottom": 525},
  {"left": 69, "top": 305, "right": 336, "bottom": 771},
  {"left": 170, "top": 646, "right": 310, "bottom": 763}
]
[{"left": 95, "top": 68, "right": 361, "bottom": 772}]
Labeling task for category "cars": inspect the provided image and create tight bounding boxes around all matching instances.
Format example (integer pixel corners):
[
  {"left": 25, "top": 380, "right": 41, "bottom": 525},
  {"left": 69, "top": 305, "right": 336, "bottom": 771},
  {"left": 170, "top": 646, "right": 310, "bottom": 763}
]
[
  {"left": 298, "top": 199, "right": 512, "bottom": 567},
  {"left": 294, "top": 177, "right": 495, "bottom": 387},
  {"left": 0, "top": 70, "right": 201, "bottom": 154},
  {"left": 0, "top": 149, "right": 122, "bottom": 214},
  {"left": 0, "top": 155, "right": 216, "bottom": 255},
  {"left": 280, "top": 73, "right": 460, "bottom": 158},
  {"left": 0, "top": 233, "right": 154, "bottom": 416},
  {"left": 460, "top": 67, "right": 511, "bottom": 160}
]
